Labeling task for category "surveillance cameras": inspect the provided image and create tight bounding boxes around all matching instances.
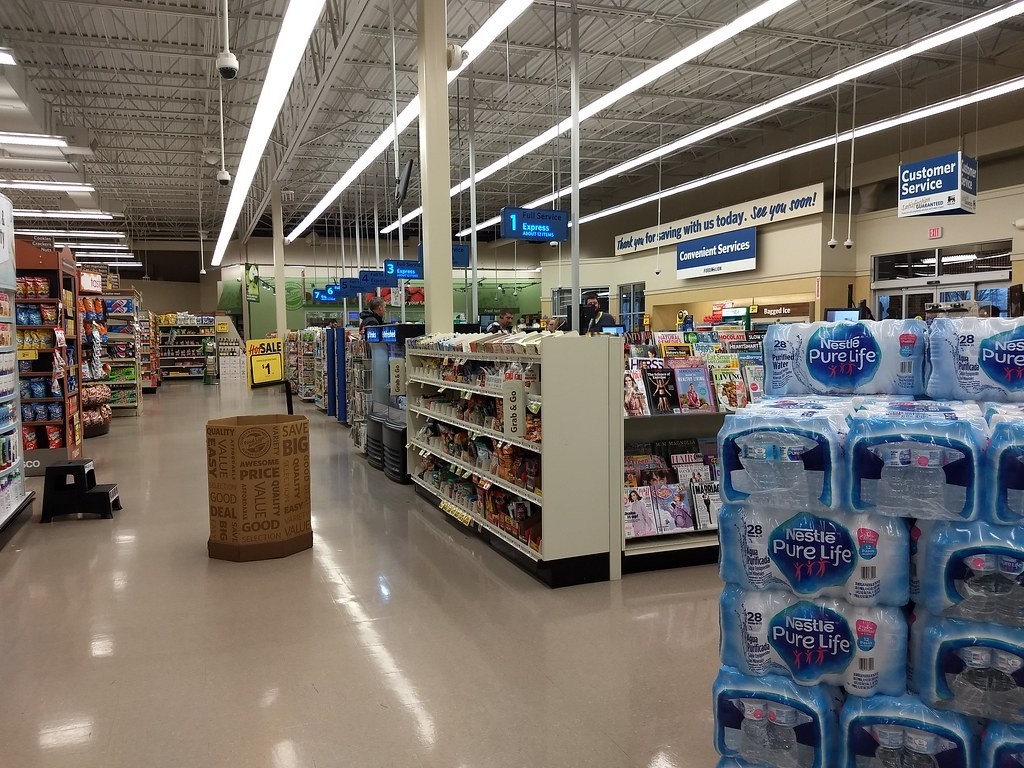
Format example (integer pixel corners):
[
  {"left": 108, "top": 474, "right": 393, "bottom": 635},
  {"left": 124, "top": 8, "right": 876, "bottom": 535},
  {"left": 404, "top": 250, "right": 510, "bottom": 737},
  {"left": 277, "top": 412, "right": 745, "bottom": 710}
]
[
  {"left": 495, "top": 298, "right": 498, "bottom": 301},
  {"left": 550, "top": 241, "right": 558, "bottom": 247},
  {"left": 654, "top": 269, "right": 661, "bottom": 275},
  {"left": 200, "top": 270, "right": 206, "bottom": 276},
  {"left": 216, "top": 58, "right": 239, "bottom": 80},
  {"left": 217, "top": 171, "right": 232, "bottom": 185},
  {"left": 828, "top": 240, "right": 837, "bottom": 248},
  {"left": 513, "top": 293, "right": 517, "bottom": 296},
  {"left": 844, "top": 240, "right": 853, "bottom": 248},
  {"left": 237, "top": 278, "right": 242, "bottom": 282}
]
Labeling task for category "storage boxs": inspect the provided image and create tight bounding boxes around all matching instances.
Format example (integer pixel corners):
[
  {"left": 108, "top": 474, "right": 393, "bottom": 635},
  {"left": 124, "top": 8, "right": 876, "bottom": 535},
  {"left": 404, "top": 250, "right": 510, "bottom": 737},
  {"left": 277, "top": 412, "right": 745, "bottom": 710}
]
[{"left": 205, "top": 414, "right": 313, "bottom": 562}]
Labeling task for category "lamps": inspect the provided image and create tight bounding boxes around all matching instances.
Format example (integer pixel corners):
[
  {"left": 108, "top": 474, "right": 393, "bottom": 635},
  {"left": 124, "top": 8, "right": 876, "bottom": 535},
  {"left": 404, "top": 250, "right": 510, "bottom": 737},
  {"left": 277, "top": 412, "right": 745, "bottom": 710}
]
[{"left": 0, "top": 0, "right": 1024, "bottom": 298}]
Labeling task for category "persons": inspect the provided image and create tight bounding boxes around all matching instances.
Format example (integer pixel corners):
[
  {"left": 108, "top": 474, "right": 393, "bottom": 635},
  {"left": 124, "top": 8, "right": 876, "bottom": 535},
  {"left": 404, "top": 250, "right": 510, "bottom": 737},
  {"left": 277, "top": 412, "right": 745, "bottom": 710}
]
[
  {"left": 545, "top": 317, "right": 566, "bottom": 333},
  {"left": 584, "top": 296, "right": 615, "bottom": 332},
  {"left": 689, "top": 471, "right": 697, "bottom": 494},
  {"left": 647, "top": 347, "right": 656, "bottom": 358},
  {"left": 628, "top": 490, "right": 656, "bottom": 535},
  {"left": 979, "top": 305, "right": 1001, "bottom": 317},
  {"left": 861, "top": 307, "right": 876, "bottom": 321},
  {"left": 486, "top": 310, "right": 514, "bottom": 334},
  {"left": 327, "top": 318, "right": 338, "bottom": 329},
  {"left": 358, "top": 296, "right": 389, "bottom": 334},
  {"left": 624, "top": 373, "right": 644, "bottom": 416},
  {"left": 649, "top": 375, "right": 673, "bottom": 414},
  {"left": 697, "top": 472, "right": 704, "bottom": 493},
  {"left": 686, "top": 382, "right": 703, "bottom": 409},
  {"left": 650, "top": 471, "right": 667, "bottom": 487}
]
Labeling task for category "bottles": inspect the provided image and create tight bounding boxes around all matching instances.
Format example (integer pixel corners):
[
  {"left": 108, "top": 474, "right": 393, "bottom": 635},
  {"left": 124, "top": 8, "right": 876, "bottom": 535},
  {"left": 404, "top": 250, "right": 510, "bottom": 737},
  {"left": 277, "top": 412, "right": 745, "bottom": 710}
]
[
  {"left": 219, "top": 337, "right": 239, "bottom": 346},
  {"left": 220, "top": 348, "right": 237, "bottom": 356},
  {"left": 713, "top": 317, "right": 1024, "bottom": 768}
]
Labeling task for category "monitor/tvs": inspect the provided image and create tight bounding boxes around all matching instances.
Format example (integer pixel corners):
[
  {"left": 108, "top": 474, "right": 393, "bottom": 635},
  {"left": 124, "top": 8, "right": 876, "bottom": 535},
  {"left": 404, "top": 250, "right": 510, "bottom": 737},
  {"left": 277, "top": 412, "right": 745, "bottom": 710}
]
[
  {"left": 601, "top": 324, "right": 625, "bottom": 334},
  {"left": 823, "top": 307, "right": 861, "bottom": 323}
]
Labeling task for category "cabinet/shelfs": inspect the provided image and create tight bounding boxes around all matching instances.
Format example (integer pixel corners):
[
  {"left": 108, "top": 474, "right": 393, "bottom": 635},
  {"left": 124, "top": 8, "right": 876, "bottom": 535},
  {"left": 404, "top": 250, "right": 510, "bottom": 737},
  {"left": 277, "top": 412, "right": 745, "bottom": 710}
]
[
  {"left": 335, "top": 321, "right": 365, "bottom": 426},
  {"left": 0, "top": 193, "right": 36, "bottom": 534},
  {"left": 285, "top": 329, "right": 299, "bottom": 396},
  {"left": 140, "top": 308, "right": 158, "bottom": 393},
  {"left": 608, "top": 315, "right": 816, "bottom": 580},
  {"left": 78, "top": 287, "right": 144, "bottom": 417},
  {"left": 296, "top": 326, "right": 324, "bottom": 402},
  {"left": 405, "top": 333, "right": 610, "bottom": 589},
  {"left": 14, "top": 233, "right": 84, "bottom": 477},
  {"left": 160, "top": 311, "right": 219, "bottom": 380},
  {"left": 312, "top": 327, "right": 332, "bottom": 414},
  {"left": 215, "top": 337, "right": 247, "bottom": 381}
]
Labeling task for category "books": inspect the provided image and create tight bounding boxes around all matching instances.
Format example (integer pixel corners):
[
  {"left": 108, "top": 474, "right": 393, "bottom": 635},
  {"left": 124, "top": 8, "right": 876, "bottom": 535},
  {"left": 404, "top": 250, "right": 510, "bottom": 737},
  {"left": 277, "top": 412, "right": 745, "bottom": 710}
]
[{"left": 624, "top": 330, "right": 764, "bottom": 536}]
